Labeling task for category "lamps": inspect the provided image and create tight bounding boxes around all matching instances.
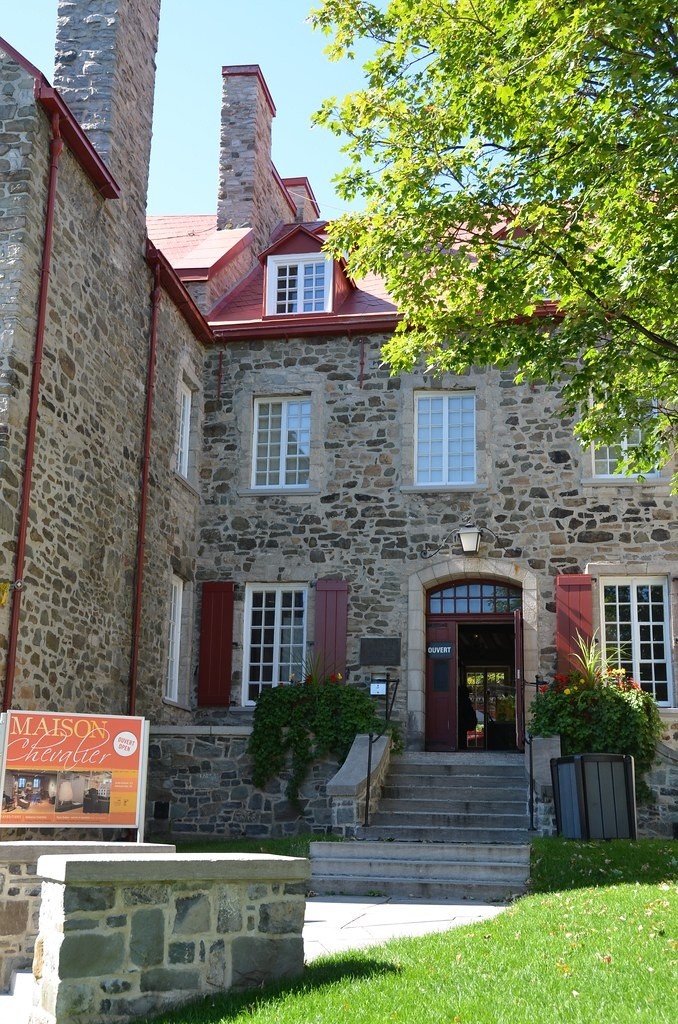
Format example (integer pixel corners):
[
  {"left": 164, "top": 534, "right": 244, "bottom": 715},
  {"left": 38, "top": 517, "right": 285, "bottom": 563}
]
[{"left": 457, "top": 515, "right": 483, "bottom": 556}]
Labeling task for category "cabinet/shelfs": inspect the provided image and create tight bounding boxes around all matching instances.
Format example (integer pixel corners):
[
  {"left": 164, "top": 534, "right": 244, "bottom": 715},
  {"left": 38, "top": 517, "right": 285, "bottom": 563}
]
[{"left": 368, "top": 672, "right": 389, "bottom": 698}]
